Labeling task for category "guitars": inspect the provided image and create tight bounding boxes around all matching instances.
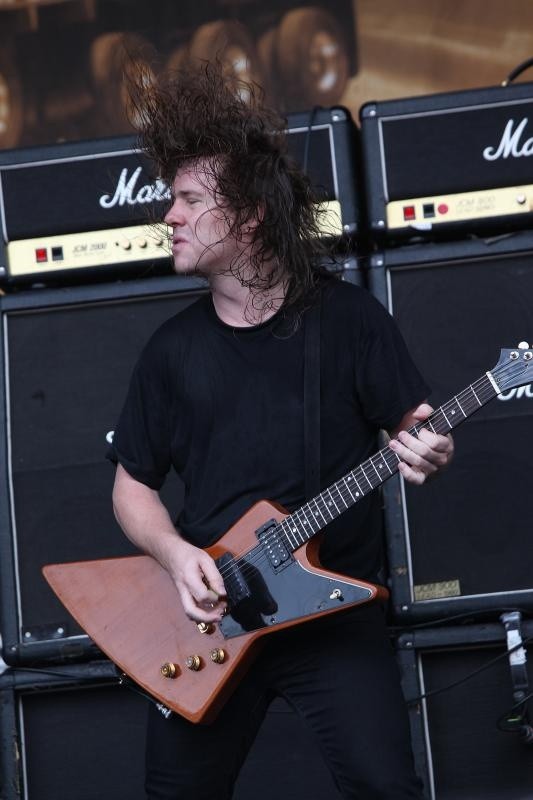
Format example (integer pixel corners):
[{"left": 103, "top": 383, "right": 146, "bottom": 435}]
[{"left": 38, "top": 341, "right": 533, "bottom": 724}]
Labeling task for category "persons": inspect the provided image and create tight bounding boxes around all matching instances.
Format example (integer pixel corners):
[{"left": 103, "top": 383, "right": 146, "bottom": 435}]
[{"left": 101, "top": 49, "right": 456, "bottom": 800}]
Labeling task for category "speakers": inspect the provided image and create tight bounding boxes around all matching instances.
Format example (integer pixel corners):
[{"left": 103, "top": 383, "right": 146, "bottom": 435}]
[{"left": 0, "top": 227, "right": 533, "bottom": 800}]
